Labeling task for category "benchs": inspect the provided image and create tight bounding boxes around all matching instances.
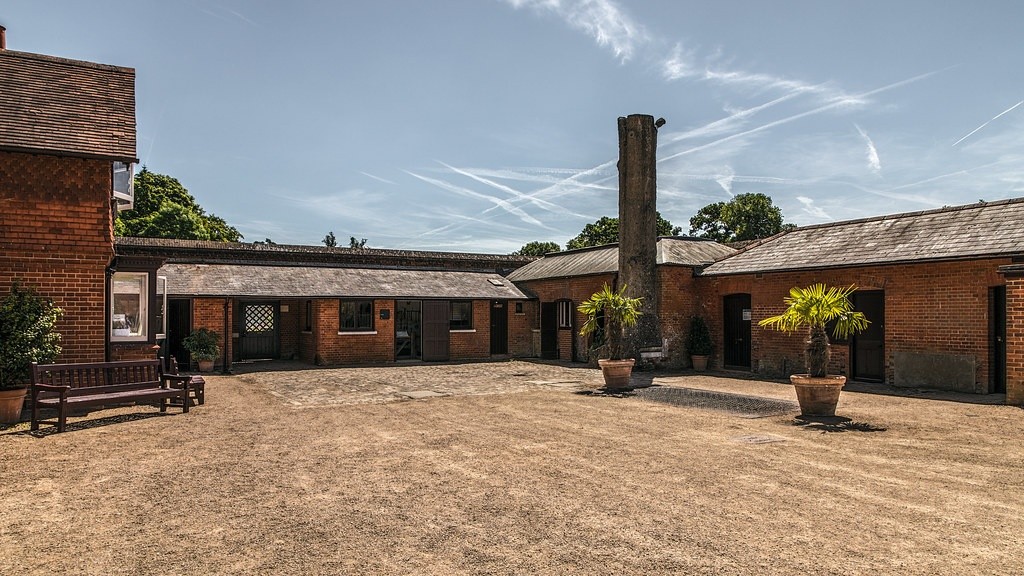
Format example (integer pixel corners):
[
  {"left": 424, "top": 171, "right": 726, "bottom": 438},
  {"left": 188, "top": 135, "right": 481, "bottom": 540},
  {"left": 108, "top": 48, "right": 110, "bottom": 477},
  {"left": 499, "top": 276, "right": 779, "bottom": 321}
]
[
  {"left": 170, "top": 355, "right": 205, "bottom": 407},
  {"left": 28, "top": 357, "right": 192, "bottom": 433}
]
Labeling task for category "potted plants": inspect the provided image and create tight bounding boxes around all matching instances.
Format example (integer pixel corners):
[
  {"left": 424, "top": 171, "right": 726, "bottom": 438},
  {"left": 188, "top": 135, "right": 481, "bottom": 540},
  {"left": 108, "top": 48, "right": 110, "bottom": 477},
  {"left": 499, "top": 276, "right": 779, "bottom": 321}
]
[
  {"left": 181, "top": 328, "right": 222, "bottom": 372},
  {"left": 0, "top": 283, "right": 67, "bottom": 426},
  {"left": 758, "top": 282, "right": 872, "bottom": 417},
  {"left": 686, "top": 316, "right": 713, "bottom": 370},
  {"left": 575, "top": 280, "right": 644, "bottom": 388}
]
